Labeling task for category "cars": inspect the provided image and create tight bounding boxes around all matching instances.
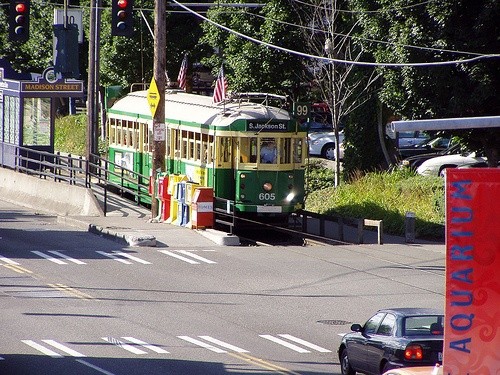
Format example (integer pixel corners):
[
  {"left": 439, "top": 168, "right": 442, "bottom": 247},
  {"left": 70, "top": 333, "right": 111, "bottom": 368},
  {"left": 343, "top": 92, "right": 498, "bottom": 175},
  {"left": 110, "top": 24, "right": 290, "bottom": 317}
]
[
  {"left": 337, "top": 307, "right": 445, "bottom": 375},
  {"left": 305, "top": 126, "right": 489, "bottom": 181},
  {"left": 382, "top": 362, "right": 444, "bottom": 375}
]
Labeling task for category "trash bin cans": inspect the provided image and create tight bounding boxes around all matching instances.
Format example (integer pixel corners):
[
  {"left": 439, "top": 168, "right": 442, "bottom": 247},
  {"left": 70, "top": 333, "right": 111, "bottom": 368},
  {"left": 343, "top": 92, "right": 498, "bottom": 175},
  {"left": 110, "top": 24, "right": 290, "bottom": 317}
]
[{"left": 150, "top": 170, "right": 215, "bottom": 229}]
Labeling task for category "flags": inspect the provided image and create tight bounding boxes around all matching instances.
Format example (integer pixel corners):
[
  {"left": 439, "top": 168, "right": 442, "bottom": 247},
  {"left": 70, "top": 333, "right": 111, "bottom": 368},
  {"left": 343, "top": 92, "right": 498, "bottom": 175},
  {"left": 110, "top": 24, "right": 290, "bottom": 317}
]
[
  {"left": 176, "top": 53, "right": 189, "bottom": 91},
  {"left": 211, "top": 63, "right": 229, "bottom": 104}
]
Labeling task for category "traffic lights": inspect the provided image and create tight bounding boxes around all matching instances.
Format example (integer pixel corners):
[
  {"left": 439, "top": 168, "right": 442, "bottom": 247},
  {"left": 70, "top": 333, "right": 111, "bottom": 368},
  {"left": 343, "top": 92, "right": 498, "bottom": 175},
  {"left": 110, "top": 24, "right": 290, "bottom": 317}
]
[
  {"left": 111, "top": 0, "right": 133, "bottom": 36},
  {"left": 9, "top": 0, "right": 31, "bottom": 41}
]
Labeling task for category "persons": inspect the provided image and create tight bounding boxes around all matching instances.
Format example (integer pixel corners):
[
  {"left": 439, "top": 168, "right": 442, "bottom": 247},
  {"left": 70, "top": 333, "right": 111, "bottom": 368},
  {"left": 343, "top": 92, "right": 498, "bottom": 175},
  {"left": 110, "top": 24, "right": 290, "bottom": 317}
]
[{"left": 260, "top": 140, "right": 277, "bottom": 164}]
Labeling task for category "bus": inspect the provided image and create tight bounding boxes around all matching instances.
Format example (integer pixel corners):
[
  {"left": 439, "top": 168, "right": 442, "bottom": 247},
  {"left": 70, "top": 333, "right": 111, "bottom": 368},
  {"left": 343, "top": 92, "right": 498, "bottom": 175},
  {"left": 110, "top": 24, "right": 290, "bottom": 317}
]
[{"left": 103, "top": 81, "right": 307, "bottom": 226}]
